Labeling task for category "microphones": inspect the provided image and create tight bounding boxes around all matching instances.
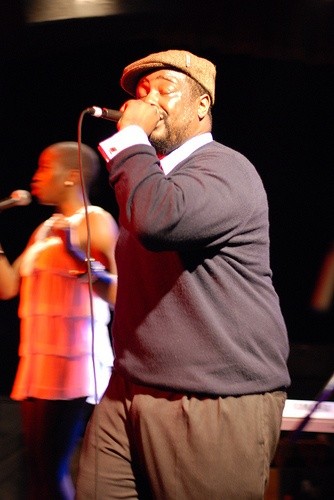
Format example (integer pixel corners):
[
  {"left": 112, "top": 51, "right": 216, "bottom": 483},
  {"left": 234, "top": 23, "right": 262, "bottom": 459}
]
[
  {"left": 91, "top": 106, "right": 123, "bottom": 122},
  {"left": 1, "top": 190, "right": 31, "bottom": 212}
]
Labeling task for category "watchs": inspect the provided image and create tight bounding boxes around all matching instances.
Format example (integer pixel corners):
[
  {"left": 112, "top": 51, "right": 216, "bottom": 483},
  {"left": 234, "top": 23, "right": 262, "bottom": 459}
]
[{"left": 86, "top": 260, "right": 105, "bottom": 272}]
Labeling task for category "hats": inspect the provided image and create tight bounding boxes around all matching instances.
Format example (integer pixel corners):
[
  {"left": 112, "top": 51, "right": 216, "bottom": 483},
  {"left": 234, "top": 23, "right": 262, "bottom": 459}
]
[{"left": 120, "top": 49, "right": 217, "bottom": 103}]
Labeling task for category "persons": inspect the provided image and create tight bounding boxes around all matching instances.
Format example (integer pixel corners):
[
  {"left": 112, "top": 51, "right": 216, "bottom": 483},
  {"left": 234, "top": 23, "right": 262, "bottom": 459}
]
[
  {"left": 0, "top": 140, "right": 120, "bottom": 500},
  {"left": 73, "top": 48, "right": 292, "bottom": 500}
]
